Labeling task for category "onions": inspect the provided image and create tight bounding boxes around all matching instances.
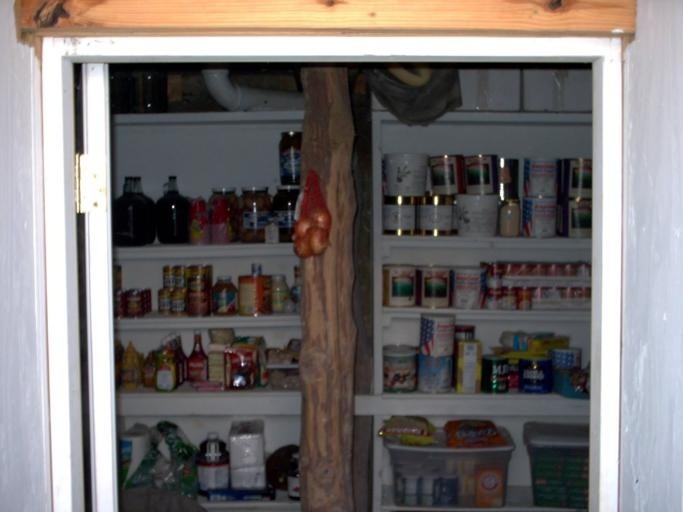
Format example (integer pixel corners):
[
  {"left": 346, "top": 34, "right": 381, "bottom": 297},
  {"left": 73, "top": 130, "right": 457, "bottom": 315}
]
[{"left": 293, "top": 211, "right": 330, "bottom": 258}]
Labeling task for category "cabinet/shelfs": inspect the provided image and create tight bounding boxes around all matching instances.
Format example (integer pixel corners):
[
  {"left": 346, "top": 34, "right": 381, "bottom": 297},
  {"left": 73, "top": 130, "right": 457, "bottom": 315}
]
[
  {"left": 374, "top": 109, "right": 593, "bottom": 511},
  {"left": 112, "top": 112, "right": 299, "bottom": 511}
]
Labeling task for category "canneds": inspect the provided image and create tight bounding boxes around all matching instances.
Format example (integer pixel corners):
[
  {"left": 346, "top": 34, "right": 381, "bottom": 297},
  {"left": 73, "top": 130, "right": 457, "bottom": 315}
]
[
  {"left": 238, "top": 275, "right": 272, "bottom": 316},
  {"left": 383, "top": 314, "right": 509, "bottom": 394},
  {"left": 383, "top": 259, "right": 592, "bottom": 311},
  {"left": 383, "top": 154, "right": 592, "bottom": 237},
  {"left": 158, "top": 264, "right": 212, "bottom": 317},
  {"left": 208, "top": 131, "right": 301, "bottom": 243}
]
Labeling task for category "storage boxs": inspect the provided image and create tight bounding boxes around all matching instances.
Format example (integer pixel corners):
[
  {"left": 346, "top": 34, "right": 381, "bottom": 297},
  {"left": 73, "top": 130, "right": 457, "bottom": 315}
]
[
  {"left": 519, "top": 422, "right": 590, "bottom": 506},
  {"left": 379, "top": 428, "right": 515, "bottom": 503},
  {"left": 454, "top": 69, "right": 524, "bottom": 112},
  {"left": 522, "top": 69, "right": 592, "bottom": 112}
]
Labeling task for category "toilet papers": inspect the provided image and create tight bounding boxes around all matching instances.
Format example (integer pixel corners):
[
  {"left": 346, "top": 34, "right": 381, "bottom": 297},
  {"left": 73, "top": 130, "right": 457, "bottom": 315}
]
[{"left": 120, "top": 429, "right": 150, "bottom": 487}]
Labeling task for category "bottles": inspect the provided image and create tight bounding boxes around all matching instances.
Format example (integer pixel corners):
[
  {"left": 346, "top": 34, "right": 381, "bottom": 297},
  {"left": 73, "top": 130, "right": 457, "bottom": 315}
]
[
  {"left": 116, "top": 329, "right": 207, "bottom": 394},
  {"left": 291, "top": 264, "right": 301, "bottom": 312},
  {"left": 287, "top": 453, "right": 299, "bottom": 500},
  {"left": 477, "top": 258, "right": 591, "bottom": 312},
  {"left": 196, "top": 431, "right": 230, "bottom": 498},
  {"left": 113, "top": 174, "right": 210, "bottom": 245}
]
